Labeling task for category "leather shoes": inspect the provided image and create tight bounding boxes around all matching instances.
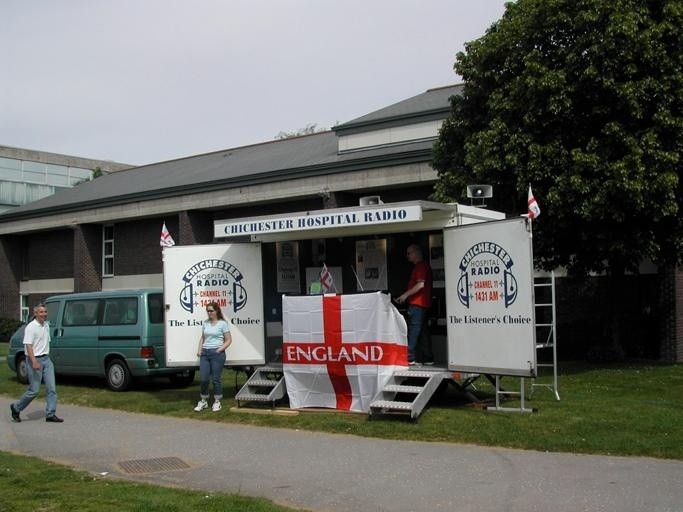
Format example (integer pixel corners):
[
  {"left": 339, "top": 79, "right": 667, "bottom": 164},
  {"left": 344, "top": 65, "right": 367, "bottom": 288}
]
[
  {"left": 45, "top": 415, "right": 64, "bottom": 423},
  {"left": 9, "top": 402, "right": 22, "bottom": 422}
]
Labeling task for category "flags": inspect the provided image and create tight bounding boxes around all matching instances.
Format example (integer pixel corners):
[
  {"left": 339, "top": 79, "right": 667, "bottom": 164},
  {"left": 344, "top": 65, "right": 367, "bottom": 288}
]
[
  {"left": 520, "top": 187, "right": 540, "bottom": 220},
  {"left": 319, "top": 264, "right": 334, "bottom": 295},
  {"left": 158, "top": 223, "right": 175, "bottom": 248}
]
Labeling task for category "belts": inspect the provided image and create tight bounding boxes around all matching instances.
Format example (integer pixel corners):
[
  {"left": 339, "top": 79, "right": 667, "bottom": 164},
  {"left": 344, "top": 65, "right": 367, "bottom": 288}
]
[{"left": 35, "top": 354, "right": 49, "bottom": 358}]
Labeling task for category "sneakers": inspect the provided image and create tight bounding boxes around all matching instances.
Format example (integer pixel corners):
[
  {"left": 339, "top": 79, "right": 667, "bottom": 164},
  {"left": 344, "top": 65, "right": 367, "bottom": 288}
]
[
  {"left": 402, "top": 355, "right": 435, "bottom": 365},
  {"left": 193, "top": 400, "right": 209, "bottom": 412},
  {"left": 212, "top": 400, "right": 223, "bottom": 412}
]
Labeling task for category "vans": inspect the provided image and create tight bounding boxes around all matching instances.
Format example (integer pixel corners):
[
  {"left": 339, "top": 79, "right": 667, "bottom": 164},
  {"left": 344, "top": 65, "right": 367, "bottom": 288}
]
[{"left": 8, "top": 286, "right": 200, "bottom": 393}]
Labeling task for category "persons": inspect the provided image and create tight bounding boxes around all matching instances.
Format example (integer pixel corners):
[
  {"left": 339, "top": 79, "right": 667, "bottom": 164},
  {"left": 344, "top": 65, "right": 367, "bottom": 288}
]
[
  {"left": 394, "top": 243, "right": 437, "bottom": 366},
  {"left": 193, "top": 302, "right": 233, "bottom": 412},
  {"left": 9, "top": 303, "right": 65, "bottom": 424}
]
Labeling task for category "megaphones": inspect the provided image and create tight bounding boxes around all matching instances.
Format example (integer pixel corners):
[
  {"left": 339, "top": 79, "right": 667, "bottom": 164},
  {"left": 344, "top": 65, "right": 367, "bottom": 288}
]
[
  {"left": 359, "top": 196, "right": 382, "bottom": 206},
  {"left": 466, "top": 184, "right": 494, "bottom": 199}
]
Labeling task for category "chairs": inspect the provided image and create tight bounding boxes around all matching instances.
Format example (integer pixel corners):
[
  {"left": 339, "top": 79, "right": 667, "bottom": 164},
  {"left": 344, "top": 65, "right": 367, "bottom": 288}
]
[
  {"left": 92, "top": 303, "right": 122, "bottom": 323},
  {"left": 68, "top": 303, "right": 89, "bottom": 325}
]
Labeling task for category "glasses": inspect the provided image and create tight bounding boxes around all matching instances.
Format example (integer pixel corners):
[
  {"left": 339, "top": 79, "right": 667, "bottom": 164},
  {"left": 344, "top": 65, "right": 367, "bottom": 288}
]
[{"left": 207, "top": 309, "right": 217, "bottom": 313}]
